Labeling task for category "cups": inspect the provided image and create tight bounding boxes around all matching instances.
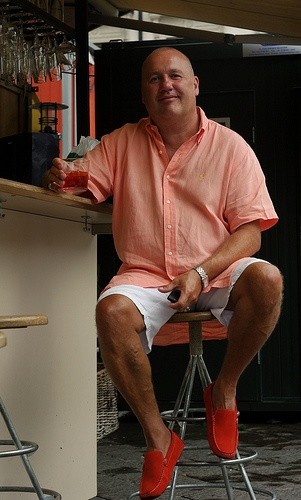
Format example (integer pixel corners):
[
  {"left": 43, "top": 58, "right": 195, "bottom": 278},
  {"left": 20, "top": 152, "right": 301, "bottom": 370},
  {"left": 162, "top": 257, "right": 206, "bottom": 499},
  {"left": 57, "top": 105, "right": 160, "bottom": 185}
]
[{"left": 61, "top": 157, "right": 90, "bottom": 193}]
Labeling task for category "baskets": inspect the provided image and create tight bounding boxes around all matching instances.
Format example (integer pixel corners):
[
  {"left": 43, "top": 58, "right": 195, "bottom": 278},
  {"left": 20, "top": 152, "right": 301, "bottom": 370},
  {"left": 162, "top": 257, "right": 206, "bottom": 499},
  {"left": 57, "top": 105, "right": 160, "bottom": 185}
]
[{"left": 96, "top": 363, "right": 119, "bottom": 440}]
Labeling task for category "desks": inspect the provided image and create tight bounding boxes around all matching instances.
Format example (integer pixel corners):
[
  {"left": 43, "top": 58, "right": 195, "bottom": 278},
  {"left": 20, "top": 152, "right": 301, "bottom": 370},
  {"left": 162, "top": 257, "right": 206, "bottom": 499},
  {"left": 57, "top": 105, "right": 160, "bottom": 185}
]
[
  {"left": 0, "top": 176, "right": 113, "bottom": 500},
  {"left": 26, "top": 102, "right": 68, "bottom": 133}
]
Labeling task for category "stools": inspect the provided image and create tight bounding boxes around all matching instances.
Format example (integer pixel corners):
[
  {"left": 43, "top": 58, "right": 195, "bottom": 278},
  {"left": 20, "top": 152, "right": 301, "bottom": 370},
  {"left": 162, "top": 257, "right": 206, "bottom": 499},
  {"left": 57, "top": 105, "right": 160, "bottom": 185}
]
[
  {"left": 0, "top": 312, "right": 62, "bottom": 500},
  {"left": 125, "top": 309, "right": 278, "bottom": 500}
]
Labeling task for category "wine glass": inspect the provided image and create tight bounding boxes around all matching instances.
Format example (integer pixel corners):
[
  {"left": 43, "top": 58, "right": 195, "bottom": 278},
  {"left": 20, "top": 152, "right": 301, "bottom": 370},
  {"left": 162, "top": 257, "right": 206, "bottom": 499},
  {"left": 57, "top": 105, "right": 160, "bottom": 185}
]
[{"left": 0, "top": 3, "right": 76, "bottom": 86}]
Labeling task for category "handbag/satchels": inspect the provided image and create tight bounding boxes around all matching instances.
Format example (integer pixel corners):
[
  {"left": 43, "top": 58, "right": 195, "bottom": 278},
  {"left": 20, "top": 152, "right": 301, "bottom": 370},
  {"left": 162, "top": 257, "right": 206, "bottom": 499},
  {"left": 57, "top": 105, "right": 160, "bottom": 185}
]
[{"left": 0, "top": 130, "right": 62, "bottom": 188}]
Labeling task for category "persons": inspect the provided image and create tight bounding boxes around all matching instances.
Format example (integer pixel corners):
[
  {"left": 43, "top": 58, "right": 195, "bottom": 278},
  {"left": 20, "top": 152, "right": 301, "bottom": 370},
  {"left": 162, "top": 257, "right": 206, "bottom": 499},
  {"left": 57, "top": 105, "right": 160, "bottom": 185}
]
[{"left": 45, "top": 47, "right": 283, "bottom": 500}]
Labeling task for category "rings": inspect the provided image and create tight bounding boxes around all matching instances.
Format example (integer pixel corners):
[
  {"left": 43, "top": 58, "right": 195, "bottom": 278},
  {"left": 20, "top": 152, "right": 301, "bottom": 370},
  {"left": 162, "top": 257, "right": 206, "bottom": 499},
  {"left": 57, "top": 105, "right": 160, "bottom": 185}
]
[
  {"left": 47, "top": 183, "right": 51, "bottom": 189},
  {"left": 184, "top": 306, "right": 191, "bottom": 313}
]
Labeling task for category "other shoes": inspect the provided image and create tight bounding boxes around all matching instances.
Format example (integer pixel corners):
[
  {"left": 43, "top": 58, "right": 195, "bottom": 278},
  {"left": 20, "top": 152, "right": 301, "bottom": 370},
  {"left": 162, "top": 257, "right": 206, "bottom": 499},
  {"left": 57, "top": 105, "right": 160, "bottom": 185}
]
[
  {"left": 205, "top": 381, "right": 239, "bottom": 458},
  {"left": 139, "top": 429, "right": 185, "bottom": 500}
]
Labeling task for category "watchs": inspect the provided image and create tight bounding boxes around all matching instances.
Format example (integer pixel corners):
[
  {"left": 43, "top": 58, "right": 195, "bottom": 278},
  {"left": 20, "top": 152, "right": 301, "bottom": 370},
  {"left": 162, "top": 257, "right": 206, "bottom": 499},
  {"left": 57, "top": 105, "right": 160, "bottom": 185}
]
[{"left": 193, "top": 266, "right": 209, "bottom": 291}]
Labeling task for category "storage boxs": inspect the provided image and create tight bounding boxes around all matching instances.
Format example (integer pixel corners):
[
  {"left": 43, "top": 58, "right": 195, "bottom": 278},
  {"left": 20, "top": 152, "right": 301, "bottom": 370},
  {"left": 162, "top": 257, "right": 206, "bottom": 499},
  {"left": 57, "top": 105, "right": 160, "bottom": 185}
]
[{"left": 0, "top": 131, "right": 60, "bottom": 187}]
